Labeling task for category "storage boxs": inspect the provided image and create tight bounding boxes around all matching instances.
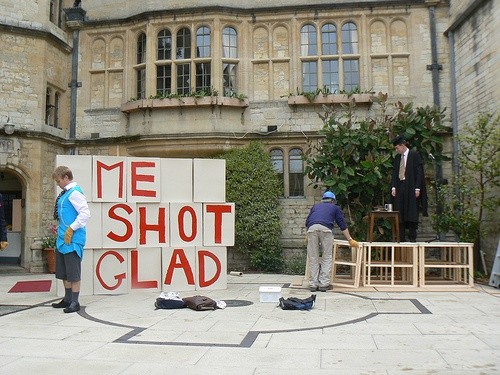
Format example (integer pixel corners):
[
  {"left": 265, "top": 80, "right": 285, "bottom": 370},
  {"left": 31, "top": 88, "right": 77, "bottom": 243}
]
[{"left": 259, "top": 286, "right": 281, "bottom": 302}]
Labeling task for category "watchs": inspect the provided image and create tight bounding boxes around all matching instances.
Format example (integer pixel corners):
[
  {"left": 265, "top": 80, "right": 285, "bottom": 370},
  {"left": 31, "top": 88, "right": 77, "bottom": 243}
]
[{"left": 348, "top": 237, "right": 352, "bottom": 241}]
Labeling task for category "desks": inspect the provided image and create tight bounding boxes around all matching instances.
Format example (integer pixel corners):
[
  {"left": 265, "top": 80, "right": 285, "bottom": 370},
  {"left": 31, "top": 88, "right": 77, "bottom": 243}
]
[{"left": 0, "top": 232, "right": 21, "bottom": 262}]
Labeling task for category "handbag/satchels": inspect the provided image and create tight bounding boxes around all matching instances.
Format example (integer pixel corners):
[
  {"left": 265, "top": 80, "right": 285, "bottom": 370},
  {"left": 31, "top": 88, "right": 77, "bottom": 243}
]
[
  {"left": 279, "top": 294, "right": 316, "bottom": 310},
  {"left": 155, "top": 298, "right": 184, "bottom": 309},
  {"left": 183, "top": 296, "right": 216, "bottom": 310}
]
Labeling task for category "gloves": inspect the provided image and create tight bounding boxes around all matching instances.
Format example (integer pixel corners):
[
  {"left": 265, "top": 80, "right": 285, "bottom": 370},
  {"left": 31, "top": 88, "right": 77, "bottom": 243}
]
[
  {"left": 349, "top": 239, "right": 358, "bottom": 247},
  {"left": 64, "top": 226, "right": 74, "bottom": 245},
  {"left": 0, "top": 241, "right": 8, "bottom": 249}
]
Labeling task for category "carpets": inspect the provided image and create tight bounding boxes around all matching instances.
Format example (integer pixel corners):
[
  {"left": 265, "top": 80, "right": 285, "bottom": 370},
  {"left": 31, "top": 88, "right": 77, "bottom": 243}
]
[{"left": 8, "top": 280, "right": 52, "bottom": 293}]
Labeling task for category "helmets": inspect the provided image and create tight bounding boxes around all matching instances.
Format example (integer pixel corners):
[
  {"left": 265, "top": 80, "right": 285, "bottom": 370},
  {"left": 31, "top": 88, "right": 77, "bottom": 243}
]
[{"left": 322, "top": 192, "right": 337, "bottom": 203}]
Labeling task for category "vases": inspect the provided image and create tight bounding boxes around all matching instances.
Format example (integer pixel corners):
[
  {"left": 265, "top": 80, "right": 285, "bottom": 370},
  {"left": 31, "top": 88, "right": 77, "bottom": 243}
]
[{"left": 44, "top": 248, "right": 56, "bottom": 274}]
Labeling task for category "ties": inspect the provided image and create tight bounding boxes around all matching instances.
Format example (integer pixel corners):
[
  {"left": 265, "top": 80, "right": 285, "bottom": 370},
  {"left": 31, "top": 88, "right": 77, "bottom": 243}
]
[
  {"left": 54, "top": 189, "right": 67, "bottom": 219},
  {"left": 399, "top": 153, "right": 406, "bottom": 180}
]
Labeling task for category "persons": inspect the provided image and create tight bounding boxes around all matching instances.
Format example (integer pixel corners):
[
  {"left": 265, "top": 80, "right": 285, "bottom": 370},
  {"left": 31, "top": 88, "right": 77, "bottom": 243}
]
[
  {"left": 389, "top": 137, "right": 428, "bottom": 242},
  {"left": 305, "top": 191, "right": 359, "bottom": 290},
  {"left": 52, "top": 166, "right": 92, "bottom": 313},
  {"left": 0, "top": 190, "right": 8, "bottom": 250}
]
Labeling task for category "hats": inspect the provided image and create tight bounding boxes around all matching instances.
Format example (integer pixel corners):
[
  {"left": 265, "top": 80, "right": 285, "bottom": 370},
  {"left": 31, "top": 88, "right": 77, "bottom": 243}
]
[{"left": 392, "top": 135, "right": 403, "bottom": 146}]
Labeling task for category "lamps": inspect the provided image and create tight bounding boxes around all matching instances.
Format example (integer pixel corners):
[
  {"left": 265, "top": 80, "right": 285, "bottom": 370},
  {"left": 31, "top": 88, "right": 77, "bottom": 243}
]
[
  {"left": 4, "top": 116, "right": 15, "bottom": 135},
  {"left": 63, "top": 0, "right": 86, "bottom": 31}
]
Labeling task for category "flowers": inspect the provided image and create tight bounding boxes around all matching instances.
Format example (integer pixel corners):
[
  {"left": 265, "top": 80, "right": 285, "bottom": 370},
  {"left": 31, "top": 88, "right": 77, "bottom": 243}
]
[{"left": 40, "top": 224, "right": 56, "bottom": 249}]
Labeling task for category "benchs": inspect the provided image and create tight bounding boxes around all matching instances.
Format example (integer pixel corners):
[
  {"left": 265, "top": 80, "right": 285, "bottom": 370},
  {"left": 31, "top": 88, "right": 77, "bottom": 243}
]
[{"left": 328, "top": 239, "right": 481, "bottom": 292}]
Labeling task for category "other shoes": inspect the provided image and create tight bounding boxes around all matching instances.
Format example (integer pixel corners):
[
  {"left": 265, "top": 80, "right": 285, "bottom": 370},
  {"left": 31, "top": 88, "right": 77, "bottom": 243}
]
[
  {"left": 410, "top": 237, "right": 415, "bottom": 242},
  {"left": 52, "top": 300, "right": 70, "bottom": 308},
  {"left": 319, "top": 284, "right": 333, "bottom": 291},
  {"left": 311, "top": 287, "right": 317, "bottom": 292},
  {"left": 64, "top": 302, "right": 80, "bottom": 313}
]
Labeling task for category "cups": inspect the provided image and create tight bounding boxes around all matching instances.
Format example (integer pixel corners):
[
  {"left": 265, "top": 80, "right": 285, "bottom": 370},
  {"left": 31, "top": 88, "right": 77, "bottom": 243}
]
[{"left": 384, "top": 204, "right": 392, "bottom": 211}]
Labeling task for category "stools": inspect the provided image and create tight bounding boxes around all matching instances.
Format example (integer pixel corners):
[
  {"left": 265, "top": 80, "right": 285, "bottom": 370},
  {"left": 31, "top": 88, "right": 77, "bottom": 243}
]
[{"left": 366, "top": 210, "right": 402, "bottom": 280}]
